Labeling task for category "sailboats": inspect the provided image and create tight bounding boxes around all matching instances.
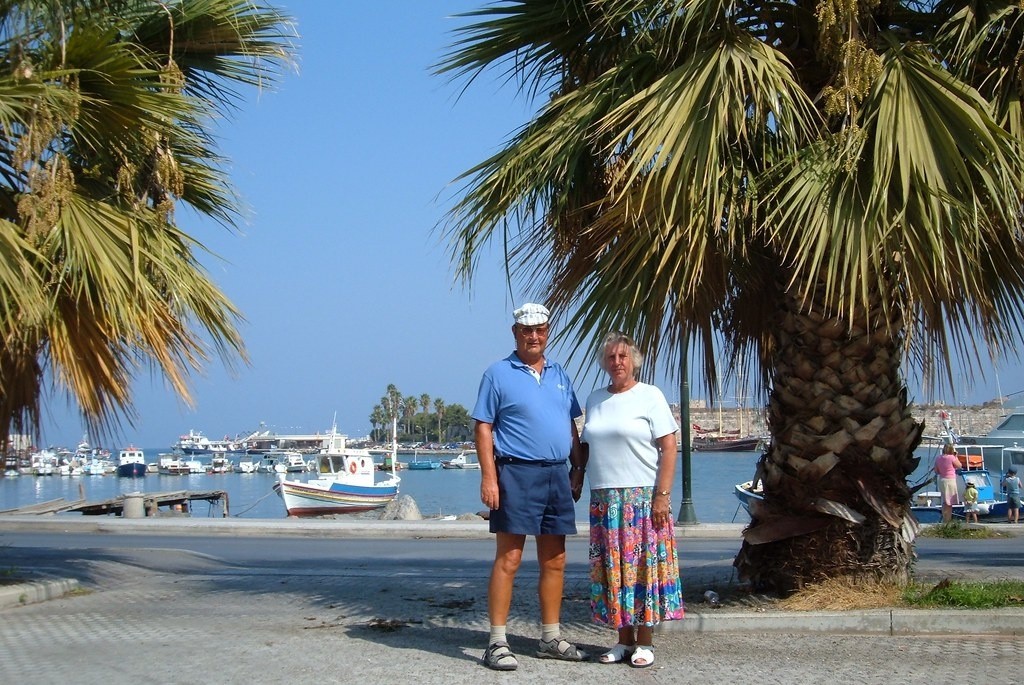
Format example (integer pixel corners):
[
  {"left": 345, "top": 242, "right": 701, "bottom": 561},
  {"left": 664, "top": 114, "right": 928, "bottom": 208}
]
[{"left": 692, "top": 344, "right": 764, "bottom": 453}]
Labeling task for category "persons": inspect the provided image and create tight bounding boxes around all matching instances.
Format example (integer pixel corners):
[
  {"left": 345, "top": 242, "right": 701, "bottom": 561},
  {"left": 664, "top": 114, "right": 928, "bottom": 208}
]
[
  {"left": 472, "top": 302, "right": 685, "bottom": 670},
  {"left": 934, "top": 443, "right": 962, "bottom": 523},
  {"left": 963, "top": 478, "right": 979, "bottom": 523},
  {"left": 1002, "top": 466, "right": 1023, "bottom": 523}
]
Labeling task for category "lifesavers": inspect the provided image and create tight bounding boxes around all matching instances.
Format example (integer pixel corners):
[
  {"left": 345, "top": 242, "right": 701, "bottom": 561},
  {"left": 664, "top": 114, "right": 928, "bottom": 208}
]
[{"left": 350, "top": 462, "right": 356, "bottom": 473}]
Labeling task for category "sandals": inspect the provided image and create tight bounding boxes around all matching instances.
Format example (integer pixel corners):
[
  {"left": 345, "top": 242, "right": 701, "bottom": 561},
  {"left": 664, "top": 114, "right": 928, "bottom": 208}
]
[
  {"left": 631, "top": 644, "right": 655, "bottom": 668},
  {"left": 536, "top": 636, "right": 591, "bottom": 661},
  {"left": 599, "top": 643, "right": 637, "bottom": 663},
  {"left": 484, "top": 641, "right": 518, "bottom": 670}
]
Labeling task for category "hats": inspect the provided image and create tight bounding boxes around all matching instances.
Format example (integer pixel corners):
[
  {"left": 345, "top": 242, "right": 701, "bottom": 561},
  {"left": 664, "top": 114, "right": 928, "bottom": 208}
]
[
  {"left": 965, "top": 477, "right": 976, "bottom": 486},
  {"left": 1009, "top": 466, "right": 1017, "bottom": 473},
  {"left": 512, "top": 303, "right": 550, "bottom": 326}
]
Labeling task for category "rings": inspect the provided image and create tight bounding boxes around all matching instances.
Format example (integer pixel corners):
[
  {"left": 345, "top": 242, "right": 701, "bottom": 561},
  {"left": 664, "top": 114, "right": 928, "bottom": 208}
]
[{"left": 662, "top": 517, "right": 665, "bottom": 519}]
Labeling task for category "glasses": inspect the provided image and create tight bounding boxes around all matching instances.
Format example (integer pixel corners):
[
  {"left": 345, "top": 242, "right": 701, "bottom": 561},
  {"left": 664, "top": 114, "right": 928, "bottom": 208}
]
[{"left": 516, "top": 327, "right": 547, "bottom": 335}]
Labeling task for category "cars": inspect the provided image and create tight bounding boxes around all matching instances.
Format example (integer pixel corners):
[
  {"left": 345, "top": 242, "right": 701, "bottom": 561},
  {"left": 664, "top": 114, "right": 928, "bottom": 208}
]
[{"left": 374, "top": 441, "right": 474, "bottom": 449}]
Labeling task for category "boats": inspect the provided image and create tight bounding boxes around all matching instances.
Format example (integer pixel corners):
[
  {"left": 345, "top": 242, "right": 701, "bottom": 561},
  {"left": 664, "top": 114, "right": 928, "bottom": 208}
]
[
  {"left": 440, "top": 452, "right": 466, "bottom": 468},
  {"left": 735, "top": 444, "right": 1023, "bottom": 522},
  {"left": 376, "top": 451, "right": 400, "bottom": 472},
  {"left": 677, "top": 442, "right": 694, "bottom": 452},
  {"left": 271, "top": 408, "right": 401, "bottom": 519},
  {"left": 924, "top": 407, "right": 1024, "bottom": 476},
  {"left": 460, "top": 463, "right": 481, "bottom": 469},
  {"left": 407, "top": 448, "right": 442, "bottom": 470},
  {"left": 2, "top": 428, "right": 316, "bottom": 479}
]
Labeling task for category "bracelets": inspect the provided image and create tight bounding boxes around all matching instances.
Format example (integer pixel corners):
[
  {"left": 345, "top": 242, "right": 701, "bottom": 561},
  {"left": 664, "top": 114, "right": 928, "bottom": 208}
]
[
  {"left": 654, "top": 490, "right": 671, "bottom": 495},
  {"left": 573, "top": 464, "right": 586, "bottom": 471}
]
